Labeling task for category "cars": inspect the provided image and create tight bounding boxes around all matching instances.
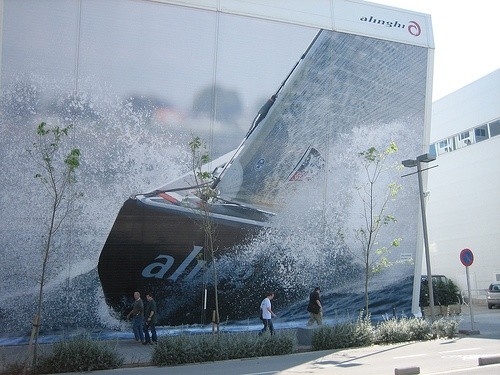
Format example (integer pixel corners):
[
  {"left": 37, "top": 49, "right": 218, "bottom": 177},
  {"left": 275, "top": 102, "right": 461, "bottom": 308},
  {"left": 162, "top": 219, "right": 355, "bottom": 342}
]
[
  {"left": 484, "top": 281, "right": 500, "bottom": 309},
  {"left": 421, "top": 275, "right": 463, "bottom": 304}
]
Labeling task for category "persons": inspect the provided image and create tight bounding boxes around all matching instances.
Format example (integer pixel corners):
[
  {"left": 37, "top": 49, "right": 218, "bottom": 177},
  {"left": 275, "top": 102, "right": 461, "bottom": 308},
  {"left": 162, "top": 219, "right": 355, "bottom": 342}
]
[
  {"left": 258, "top": 293, "right": 277, "bottom": 335},
  {"left": 142, "top": 293, "right": 158, "bottom": 345},
  {"left": 127, "top": 292, "right": 144, "bottom": 343},
  {"left": 307, "top": 287, "right": 323, "bottom": 326}
]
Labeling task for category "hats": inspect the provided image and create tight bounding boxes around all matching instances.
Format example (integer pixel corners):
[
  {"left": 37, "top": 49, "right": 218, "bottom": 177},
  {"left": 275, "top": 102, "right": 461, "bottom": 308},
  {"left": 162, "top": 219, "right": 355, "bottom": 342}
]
[{"left": 315, "top": 287, "right": 321, "bottom": 292}]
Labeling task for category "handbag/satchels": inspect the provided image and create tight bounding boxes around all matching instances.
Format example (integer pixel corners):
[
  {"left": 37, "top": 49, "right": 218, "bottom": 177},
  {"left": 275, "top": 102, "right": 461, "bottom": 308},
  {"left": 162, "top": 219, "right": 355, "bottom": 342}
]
[{"left": 307, "top": 303, "right": 320, "bottom": 314}]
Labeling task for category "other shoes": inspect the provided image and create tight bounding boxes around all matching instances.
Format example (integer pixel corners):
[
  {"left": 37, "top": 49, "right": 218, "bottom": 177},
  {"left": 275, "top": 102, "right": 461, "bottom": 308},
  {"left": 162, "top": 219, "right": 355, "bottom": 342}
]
[
  {"left": 142, "top": 340, "right": 150, "bottom": 344},
  {"left": 152, "top": 340, "right": 158, "bottom": 343}
]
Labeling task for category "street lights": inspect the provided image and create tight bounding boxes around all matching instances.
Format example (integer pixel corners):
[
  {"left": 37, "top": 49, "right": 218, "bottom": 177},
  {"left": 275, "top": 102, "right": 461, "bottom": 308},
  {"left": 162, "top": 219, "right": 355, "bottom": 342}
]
[{"left": 401, "top": 153, "right": 437, "bottom": 339}]
